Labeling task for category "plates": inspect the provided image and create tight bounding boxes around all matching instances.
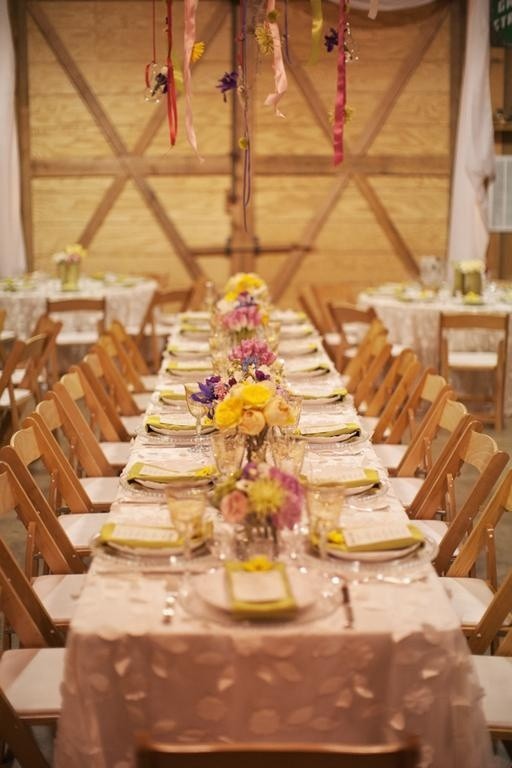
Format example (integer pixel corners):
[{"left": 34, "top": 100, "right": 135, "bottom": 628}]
[
  {"left": 132, "top": 474, "right": 218, "bottom": 491},
  {"left": 172, "top": 350, "right": 210, "bottom": 359},
  {"left": 169, "top": 369, "right": 210, "bottom": 377},
  {"left": 160, "top": 395, "right": 189, "bottom": 407},
  {"left": 283, "top": 370, "right": 331, "bottom": 379},
  {"left": 107, "top": 535, "right": 210, "bottom": 558},
  {"left": 301, "top": 394, "right": 341, "bottom": 406},
  {"left": 299, "top": 431, "right": 358, "bottom": 443},
  {"left": 196, "top": 568, "right": 319, "bottom": 612},
  {"left": 317, "top": 543, "right": 422, "bottom": 561},
  {"left": 148, "top": 425, "right": 220, "bottom": 437},
  {"left": 312, "top": 484, "right": 377, "bottom": 496},
  {"left": 176, "top": 569, "right": 346, "bottom": 625}
]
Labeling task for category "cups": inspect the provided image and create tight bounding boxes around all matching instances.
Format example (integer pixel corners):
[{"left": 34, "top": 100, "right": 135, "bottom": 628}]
[
  {"left": 277, "top": 396, "right": 302, "bottom": 434},
  {"left": 305, "top": 488, "right": 344, "bottom": 566},
  {"left": 210, "top": 430, "right": 247, "bottom": 477},
  {"left": 270, "top": 435, "right": 309, "bottom": 475}
]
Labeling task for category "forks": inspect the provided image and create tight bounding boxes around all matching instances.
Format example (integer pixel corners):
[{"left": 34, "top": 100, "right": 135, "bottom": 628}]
[{"left": 162, "top": 575, "right": 179, "bottom": 617}]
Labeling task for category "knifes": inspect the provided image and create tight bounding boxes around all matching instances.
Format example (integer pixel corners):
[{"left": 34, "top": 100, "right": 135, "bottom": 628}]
[{"left": 341, "top": 581, "right": 354, "bottom": 627}]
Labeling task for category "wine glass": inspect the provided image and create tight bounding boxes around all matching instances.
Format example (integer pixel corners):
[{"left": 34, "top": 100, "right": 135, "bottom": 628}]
[
  {"left": 183, "top": 382, "right": 211, "bottom": 453},
  {"left": 164, "top": 487, "right": 206, "bottom": 567}
]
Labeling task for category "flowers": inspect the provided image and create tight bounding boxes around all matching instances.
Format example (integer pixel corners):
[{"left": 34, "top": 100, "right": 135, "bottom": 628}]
[
  {"left": 49, "top": 242, "right": 89, "bottom": 265},
  {"left": 450, "top": 260, "right": 485, "bottom": 273}
]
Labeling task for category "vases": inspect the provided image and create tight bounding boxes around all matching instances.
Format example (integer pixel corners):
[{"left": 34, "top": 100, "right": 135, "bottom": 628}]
[{"left": 54, "top": 258, "right": 81, "bottom": 291}]
